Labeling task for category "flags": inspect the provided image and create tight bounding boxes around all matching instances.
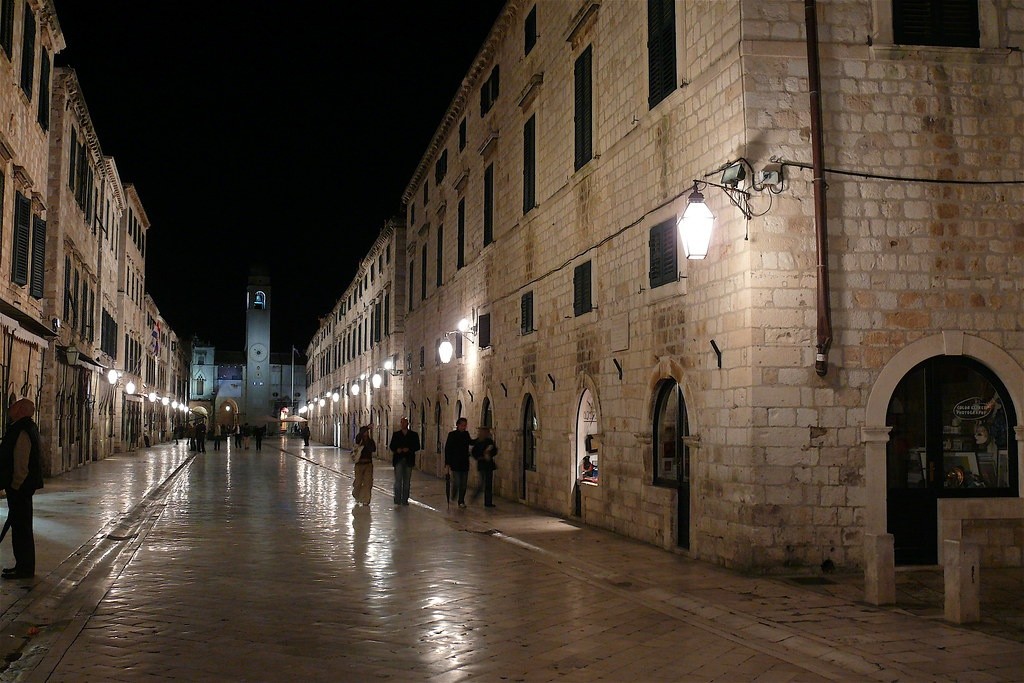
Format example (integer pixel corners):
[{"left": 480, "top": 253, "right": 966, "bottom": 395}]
[{"left": 147, "top": 323, "right": 160, "bottom": 360}]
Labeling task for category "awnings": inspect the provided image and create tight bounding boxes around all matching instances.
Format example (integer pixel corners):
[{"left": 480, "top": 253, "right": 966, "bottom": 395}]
[
  {"left": 57, "top": 344, "right": 109, "bottom": 370},
  {"left": 0, "top": 298, "right": 59, "bottom": 338}
]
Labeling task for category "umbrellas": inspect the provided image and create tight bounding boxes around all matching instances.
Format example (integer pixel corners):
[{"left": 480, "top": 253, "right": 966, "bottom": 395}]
[
  {"left": 280, "top": 414, "right": 309, "bottom": 422},
  {"left": 446, "top": 466, "right": 451, "bottom": 509}
]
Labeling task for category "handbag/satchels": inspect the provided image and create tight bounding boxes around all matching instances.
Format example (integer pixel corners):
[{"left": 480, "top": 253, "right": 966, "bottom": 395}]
[{"left": 350, "top": 438, "right": 365, "bottom": 463}]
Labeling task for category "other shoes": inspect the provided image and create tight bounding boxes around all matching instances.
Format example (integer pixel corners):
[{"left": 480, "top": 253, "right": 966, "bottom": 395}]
[
  {"left": 487, "top": 503, "right": 496, "bottom": 507},
  {"left": 3, "top": 567, "right": 16, "bottom": 572},
  {"left": 459, "top": 503, "right": 467, "bottom": 508},
  {"left": 1, "top": 570, "right": 33, "bottom": 578},
  {"left": 403, "top": 500, "right": 409, "bottom": 505}
]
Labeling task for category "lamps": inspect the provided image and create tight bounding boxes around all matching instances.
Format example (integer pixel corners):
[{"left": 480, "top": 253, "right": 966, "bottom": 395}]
[
  {"left": 103, "top": 368, "right": 119, "bottom": 385},
  {"left": 56, "top": 339, "right": 80, "bottom": 366},
  {"left": 676, "top": 179, "right": 751, "bottom": 260},
  {"left": 439, "top": 330, "right": 473, "bottom": 363}
]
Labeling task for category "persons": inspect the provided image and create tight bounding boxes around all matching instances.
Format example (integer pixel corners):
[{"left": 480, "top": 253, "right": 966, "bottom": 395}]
[
  {"left": 350, "top": 423, "right": 377, "bottom": 506},
  {"left": 172, "top": 420, "right": 206, "bottom": 454},
  {"left": 444, "top": 418, "right": 499, "bottom": 509},
  {"left": 213, "top": 422, "right": 221, "bottom": 451},
  {"left": 227, "top": 423, "right": 263, "bottom": 451},
  {"left": 294, "top": 424, "right": 297, "bottom": 432},
  {"left": 301, "top": 424, "right": 311, "bottom": 446},
  {"left": 143, "top": 424, "right": 151, "bottom": 448},
  {"left": 389, "top": 418, "right": 420, "bottom": 506},
  {"left": 1, "top": 398, "right": 44, "bottom": 579}
]
